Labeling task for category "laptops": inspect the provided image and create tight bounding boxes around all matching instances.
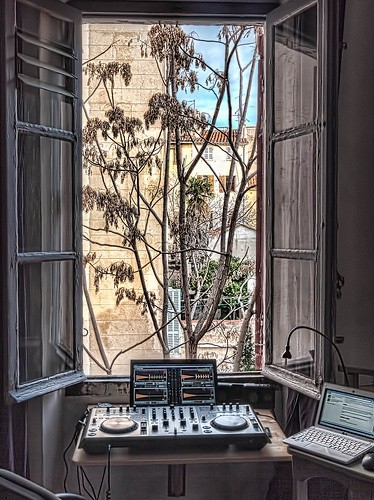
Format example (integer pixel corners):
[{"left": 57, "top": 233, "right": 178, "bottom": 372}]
[{"left": 283, "top": 381, "right": 374, "bottom": 466}]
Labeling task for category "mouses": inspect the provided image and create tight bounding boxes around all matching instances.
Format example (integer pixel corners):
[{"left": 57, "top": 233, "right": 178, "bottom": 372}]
[{"left": 361, "top": 453, "right": 374, "bottom": 470}]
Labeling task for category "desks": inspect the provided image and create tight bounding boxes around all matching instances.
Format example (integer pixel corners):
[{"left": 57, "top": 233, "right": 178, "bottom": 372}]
[
  {"left": 71, "top": 404, "right": 292, "bottom": 500},
  {"left": 287, "top": 447, "right": 374, "bottom": 500}
]
